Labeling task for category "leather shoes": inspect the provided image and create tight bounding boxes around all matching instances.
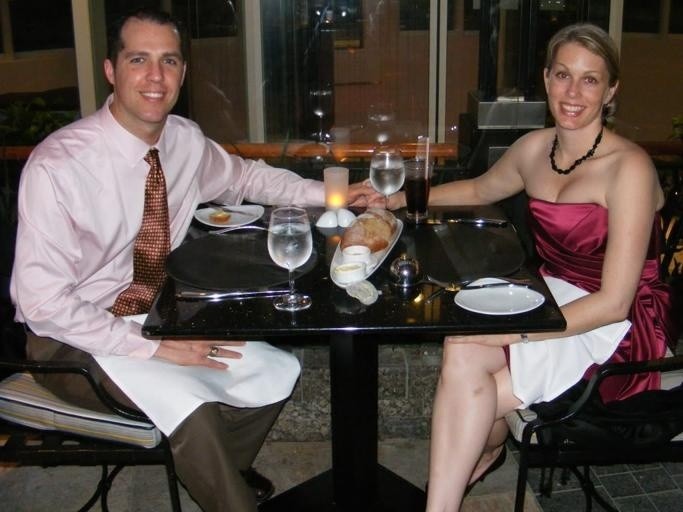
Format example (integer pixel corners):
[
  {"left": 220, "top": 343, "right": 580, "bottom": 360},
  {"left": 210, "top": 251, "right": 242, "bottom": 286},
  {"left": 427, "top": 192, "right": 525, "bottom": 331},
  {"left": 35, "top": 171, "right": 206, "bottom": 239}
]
[{"left": 233, "top": 465, "right": 275, "bottom": 507}]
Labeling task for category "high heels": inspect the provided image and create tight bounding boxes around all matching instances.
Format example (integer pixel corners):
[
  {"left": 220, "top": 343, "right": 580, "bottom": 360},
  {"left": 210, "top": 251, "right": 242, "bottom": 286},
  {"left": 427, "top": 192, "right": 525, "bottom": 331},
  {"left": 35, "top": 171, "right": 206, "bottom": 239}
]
[{"left": 421, "top": 446, "right": 510, "bottom": 498}]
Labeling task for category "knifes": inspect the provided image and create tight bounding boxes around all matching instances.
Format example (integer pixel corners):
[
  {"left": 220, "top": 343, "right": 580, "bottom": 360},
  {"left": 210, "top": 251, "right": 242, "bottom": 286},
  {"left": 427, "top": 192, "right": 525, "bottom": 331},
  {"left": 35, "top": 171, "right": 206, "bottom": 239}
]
[
  {"left": 417, "top": 219, "right": 506, "bottom": 226},
  {"left": 174, "top": 289, "right": 298, "bottom": 299}
]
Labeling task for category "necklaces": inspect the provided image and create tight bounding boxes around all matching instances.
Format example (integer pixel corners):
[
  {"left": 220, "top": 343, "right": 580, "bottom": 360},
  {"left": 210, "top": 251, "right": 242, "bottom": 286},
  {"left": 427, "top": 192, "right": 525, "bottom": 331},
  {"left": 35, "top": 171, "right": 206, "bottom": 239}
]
[{"left": 549, "top": 125, "right": 604, "bottom": 175}]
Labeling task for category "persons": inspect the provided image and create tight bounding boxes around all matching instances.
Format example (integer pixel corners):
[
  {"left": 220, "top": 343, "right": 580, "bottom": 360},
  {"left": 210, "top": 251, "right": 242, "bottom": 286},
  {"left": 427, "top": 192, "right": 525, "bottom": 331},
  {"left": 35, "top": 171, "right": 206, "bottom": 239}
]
[
  {"left": 8, "top": 9, "right": 384, "bottom": 512},
  {"left": 364, "top": 22, "right": 671, "bottom": 512}
]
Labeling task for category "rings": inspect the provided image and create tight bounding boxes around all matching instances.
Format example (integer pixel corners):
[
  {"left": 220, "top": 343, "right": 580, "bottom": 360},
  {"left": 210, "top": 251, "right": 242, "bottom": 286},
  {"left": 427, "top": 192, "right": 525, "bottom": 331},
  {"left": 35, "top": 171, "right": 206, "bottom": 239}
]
[{"left": 210, "top": 346, "right": 218, "bottom": 357}]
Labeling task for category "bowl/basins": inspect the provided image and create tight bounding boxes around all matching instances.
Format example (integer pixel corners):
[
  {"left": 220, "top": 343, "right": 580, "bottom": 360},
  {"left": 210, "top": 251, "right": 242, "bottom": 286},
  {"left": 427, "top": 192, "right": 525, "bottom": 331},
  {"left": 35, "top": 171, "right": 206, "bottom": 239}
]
[
  {"left": 341, "top": 244, "right": 371, "bottom": 264},
  {"left": 334, "top": 260, "right": 366, "bottom": 283}
]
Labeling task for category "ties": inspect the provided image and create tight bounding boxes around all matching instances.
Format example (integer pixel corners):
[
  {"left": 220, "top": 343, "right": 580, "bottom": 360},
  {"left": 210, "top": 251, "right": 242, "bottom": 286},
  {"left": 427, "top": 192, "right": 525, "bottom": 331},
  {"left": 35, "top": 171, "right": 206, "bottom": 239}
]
[{"left": 103, "top": 146, "right": 173, "bottom": 319}]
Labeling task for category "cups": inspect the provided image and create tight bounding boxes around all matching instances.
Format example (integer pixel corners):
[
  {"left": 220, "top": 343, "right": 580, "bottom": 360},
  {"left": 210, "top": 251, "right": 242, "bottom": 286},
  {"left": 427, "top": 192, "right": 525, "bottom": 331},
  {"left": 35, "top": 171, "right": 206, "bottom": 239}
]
[
  {"left": 403, "top": 159, "right": 431, "bottom": 220},
  {"left": 331, "top": 128, "right": 351, "bottom": 164},
  {"left": 323, "top": 166, "right": 350, "bottom": 211}
]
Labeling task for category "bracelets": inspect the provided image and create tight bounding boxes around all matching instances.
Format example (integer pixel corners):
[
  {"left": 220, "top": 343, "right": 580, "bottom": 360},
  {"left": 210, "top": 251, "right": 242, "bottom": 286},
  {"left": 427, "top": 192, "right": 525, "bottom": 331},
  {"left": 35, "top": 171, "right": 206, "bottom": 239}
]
[{"left": 520, "top": 333, "right": 528, "bottom": 343}]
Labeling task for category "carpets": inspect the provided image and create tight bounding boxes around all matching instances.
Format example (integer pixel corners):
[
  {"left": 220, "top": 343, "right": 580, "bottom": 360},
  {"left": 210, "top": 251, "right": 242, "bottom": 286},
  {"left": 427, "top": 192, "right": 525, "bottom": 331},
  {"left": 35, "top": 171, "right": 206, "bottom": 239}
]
[{"left": 0, "top": 431, "right": 545, "bottom": 512}]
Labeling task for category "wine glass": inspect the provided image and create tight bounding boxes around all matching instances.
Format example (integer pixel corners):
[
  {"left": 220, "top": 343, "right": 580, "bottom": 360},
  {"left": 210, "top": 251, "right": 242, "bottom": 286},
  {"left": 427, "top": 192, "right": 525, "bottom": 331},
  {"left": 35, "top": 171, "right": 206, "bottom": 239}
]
[
  {"left": 369, "top": 147, "right": 405, "bottom": 209},
  {"left": 308, "top": 80, "right": 332, "bottom": 140},
  {"left": 267, "top": 206, "right": 313, "bottom": 312},
  {"left": 368, "top": 103, "right": 394, "bottom": 143}
]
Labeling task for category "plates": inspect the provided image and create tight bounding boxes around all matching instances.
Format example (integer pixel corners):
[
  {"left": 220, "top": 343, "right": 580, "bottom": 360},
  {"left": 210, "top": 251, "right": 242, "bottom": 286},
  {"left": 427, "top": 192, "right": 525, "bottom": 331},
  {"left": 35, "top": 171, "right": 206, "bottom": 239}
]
[
  {"left": 453, "top": 276, "right": 545, "bottom": 317},
  {"left": 330, "top": 218, "right": 404, "bottom": 288},
  {"left": 401, "top": 220, "right": 526, "bottom": 280},
  {"left": 193, "top": 204, "right": 265, "bottom": 229},
  {"left": 164, "top": 232, "right": 318, "bottom": 291}
]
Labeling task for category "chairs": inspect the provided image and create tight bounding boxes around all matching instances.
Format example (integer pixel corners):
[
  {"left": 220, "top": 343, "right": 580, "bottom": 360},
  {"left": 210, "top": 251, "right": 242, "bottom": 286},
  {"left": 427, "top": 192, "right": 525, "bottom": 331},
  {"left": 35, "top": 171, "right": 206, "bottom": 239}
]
[
  {"left": 1, "top": 155, "right": 184, "bottom": 512},
  {"left": 514, "top": 208, "right": 682, "bottom": 511}
]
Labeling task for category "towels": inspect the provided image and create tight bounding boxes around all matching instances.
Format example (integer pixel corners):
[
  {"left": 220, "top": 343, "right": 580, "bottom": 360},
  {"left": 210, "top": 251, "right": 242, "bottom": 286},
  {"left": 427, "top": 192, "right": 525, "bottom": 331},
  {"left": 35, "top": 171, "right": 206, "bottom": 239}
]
[
  {"left": 90, "top": 313, "right": 303, "bottom": 439},
  {"left": 508, "top": 277, "right": 632, "bottom": 412}
]
